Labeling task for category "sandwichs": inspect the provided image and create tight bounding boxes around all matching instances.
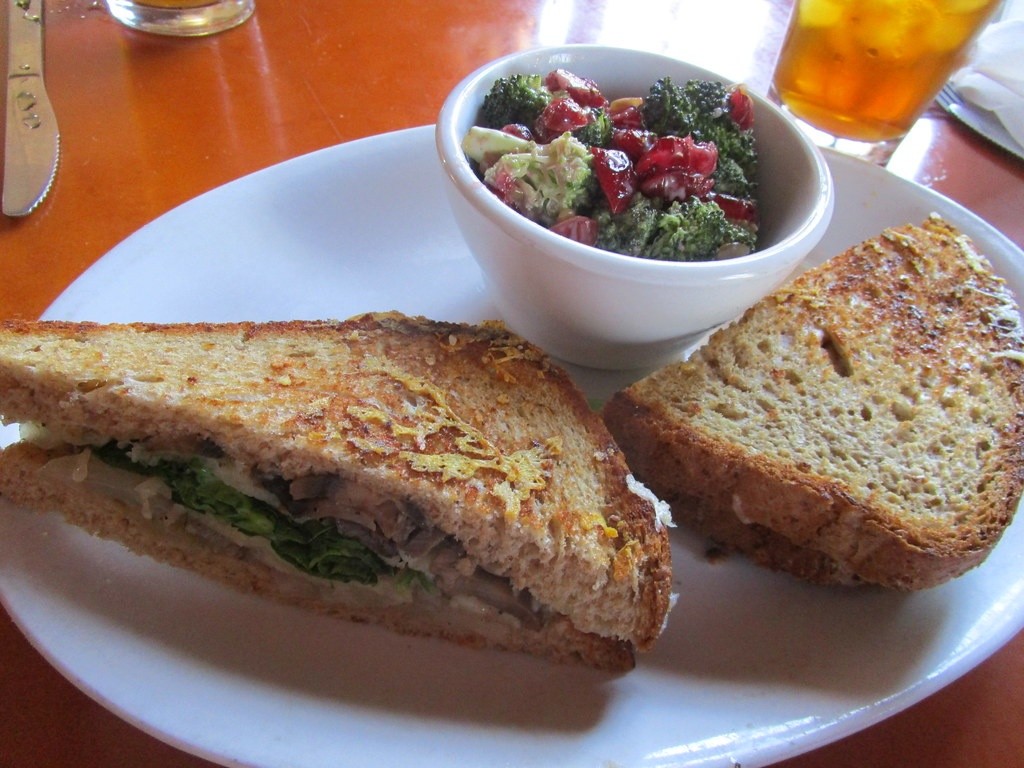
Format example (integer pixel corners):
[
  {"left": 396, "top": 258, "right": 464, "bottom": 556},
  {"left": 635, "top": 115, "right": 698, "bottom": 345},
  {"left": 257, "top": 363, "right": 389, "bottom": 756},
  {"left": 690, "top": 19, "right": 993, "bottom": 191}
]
[{"left": 0, "top": 210, "right": 1023, "bottom": 674}]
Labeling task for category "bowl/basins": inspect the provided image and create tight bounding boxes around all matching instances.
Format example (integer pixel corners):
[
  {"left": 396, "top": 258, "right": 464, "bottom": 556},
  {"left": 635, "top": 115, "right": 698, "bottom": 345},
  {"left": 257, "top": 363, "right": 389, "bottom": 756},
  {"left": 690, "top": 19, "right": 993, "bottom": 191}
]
[{"left": 435, "top": 43, "right": 834, "bottom": 375}]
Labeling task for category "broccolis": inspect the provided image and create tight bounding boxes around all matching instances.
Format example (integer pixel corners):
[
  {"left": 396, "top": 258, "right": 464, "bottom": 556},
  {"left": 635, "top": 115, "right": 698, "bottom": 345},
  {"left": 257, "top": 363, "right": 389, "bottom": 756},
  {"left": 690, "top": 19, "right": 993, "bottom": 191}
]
[{"left": 464, "top": 63, "right": 762, "bottom": 260}]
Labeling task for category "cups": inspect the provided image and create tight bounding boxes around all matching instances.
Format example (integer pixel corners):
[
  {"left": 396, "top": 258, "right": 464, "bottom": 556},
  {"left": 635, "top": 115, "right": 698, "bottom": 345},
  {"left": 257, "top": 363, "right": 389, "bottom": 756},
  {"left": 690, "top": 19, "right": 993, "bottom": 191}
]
[
  {"left": 765, "top": 0, "right": 1003, "bottom": 167},
  {"left": 106, "top": 0, "right": 255, "bottom": 37}
]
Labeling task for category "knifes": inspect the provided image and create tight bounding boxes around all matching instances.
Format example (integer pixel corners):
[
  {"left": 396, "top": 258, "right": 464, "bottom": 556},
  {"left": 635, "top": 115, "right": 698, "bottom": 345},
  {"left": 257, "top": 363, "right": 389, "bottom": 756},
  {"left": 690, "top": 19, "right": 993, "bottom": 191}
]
[{"left": 3, "top": 0, "right": 61, "bottom": 216}]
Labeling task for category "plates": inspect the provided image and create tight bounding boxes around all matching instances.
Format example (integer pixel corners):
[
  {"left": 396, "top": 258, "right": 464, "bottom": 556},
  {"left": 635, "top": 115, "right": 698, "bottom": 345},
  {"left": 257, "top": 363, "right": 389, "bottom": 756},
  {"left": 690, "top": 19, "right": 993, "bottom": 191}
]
[{"left": 3, "top": 121, "right": 1022, "bottom": 767}]
